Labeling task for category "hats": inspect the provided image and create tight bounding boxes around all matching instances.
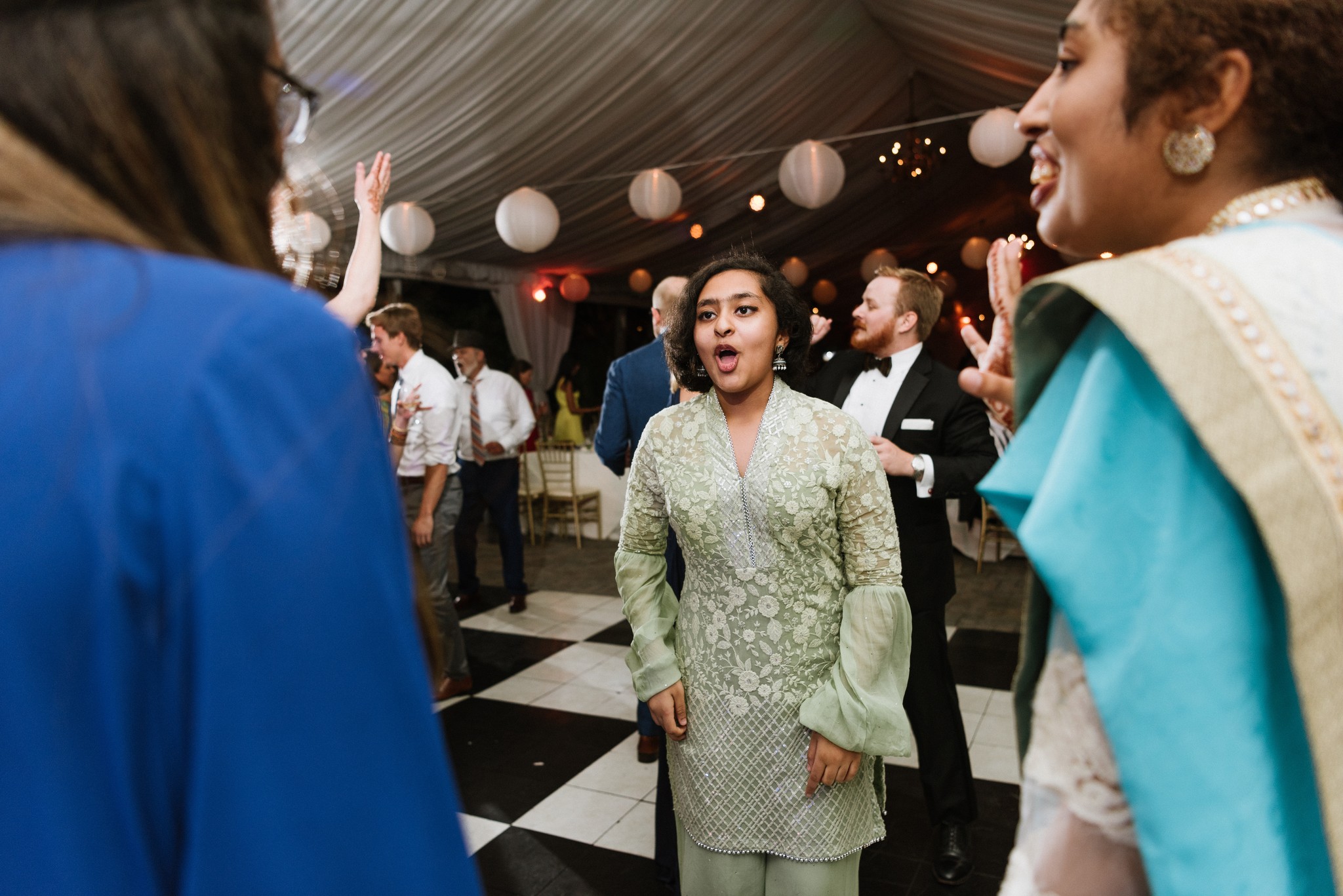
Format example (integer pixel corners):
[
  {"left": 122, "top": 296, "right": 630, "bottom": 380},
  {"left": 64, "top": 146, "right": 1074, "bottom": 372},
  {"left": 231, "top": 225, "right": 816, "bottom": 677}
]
[{"left": 443, "top": 330, "right": 493, "bottom": 358}]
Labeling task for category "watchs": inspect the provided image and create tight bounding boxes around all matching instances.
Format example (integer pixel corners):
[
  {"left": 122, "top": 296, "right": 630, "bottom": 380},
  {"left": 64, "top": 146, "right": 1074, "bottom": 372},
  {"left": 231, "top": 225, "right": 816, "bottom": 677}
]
[{"left": 912, "top": 454, "right": 924, "bottom": 479}]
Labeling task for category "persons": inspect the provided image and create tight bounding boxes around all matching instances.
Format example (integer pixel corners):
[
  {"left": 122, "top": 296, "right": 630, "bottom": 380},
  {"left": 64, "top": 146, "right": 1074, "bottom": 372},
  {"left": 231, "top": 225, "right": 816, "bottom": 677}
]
[
  {"left": 446, "top": 329, "right": 537, "bottom": 615},
  {"left": 504, "top": 359, "right": 545, "bottom": 453},
  {"left": 957, "top": 1, "right": 1343, "bottom": 896},
  {"left": 363, "top": 300, "right": 476, "bottom": 699},
  {"left": 0, "top": 0, "right": 484, "bottom": 896},
  {"left": 551, "top": 355, "right": 601, "bottom": 448},
  {"left": 613, "top": 255, "right": 918, "bottom": 896},
  {"left": 320, "top": 152, "right": 393, "bottom": 326},
  {"left": 594, "top": 276, "right": 705, "bottom": 763},
  {"left": 803, "top": 267, "right": 1001, "bottom": 885}
]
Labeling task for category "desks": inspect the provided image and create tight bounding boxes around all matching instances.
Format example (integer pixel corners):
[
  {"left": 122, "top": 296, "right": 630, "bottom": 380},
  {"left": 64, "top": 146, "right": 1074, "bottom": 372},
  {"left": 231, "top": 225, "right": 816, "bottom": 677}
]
[{"left": 519, "top": 448, "right": 630, "bottom": 542}]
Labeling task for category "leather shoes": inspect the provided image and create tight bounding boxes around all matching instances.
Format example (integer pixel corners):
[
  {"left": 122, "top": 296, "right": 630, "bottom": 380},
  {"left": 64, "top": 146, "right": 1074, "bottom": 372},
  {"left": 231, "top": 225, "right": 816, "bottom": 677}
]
[{"left": 936, "top": 825, "right": 974, "bottom": 883}]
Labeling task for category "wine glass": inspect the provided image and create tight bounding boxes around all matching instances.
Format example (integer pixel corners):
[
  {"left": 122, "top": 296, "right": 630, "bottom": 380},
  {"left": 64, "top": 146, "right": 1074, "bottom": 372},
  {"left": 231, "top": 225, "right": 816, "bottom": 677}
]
[{"left": 399, "top": 379, "right": 424, "bottom": 430}]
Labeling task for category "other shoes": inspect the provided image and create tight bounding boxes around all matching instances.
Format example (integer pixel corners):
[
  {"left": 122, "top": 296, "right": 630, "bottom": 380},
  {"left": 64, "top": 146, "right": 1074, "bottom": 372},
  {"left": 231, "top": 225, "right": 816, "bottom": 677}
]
[
  {"left": 435, "top": 675, "right": 472, "bottom": 700},
  {"left": 638, "top": 735, "right": 660, "bottom": 764},
  {"left": 455, "top": 592, "right": 478, "bottom": 605},
  {"left": 508, "top": 594, "right": 529, "bottom": 611}
]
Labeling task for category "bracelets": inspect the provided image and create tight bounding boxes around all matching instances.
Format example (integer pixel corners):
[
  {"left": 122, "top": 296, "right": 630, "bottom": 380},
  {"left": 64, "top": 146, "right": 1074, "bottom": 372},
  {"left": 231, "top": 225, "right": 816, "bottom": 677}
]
[
  {"left": 391, "top": 419, "right": 408, "bottom": 433},
  {"left": 390, "top": 433, "right": 406, "bottom": 445}
]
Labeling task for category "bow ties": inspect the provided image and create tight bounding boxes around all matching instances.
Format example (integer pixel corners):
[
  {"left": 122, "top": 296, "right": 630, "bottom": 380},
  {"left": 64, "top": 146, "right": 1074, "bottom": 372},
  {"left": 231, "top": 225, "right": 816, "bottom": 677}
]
[{"left": 863, "top": 355, "right": 893, "bottom": 379}]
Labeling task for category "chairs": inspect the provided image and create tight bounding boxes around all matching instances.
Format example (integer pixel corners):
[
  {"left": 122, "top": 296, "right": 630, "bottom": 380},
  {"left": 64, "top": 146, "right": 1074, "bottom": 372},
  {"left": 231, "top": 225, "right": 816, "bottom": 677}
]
[
  {"left": 519, "top": 441, "right": 543, "bottom": 549},
  {"left": 535, "top": 442, "right": 602, "bottom": 549}
]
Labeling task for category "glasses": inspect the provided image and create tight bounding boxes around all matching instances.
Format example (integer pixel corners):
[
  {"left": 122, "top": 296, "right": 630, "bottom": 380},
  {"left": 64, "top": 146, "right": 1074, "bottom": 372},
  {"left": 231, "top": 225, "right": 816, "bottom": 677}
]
[{"left": 258, "top": 60, "right": 320, "bottom": 150}]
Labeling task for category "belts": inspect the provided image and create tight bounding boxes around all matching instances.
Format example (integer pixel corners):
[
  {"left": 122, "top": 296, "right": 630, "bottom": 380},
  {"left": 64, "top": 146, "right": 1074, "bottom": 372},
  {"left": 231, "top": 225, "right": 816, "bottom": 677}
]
[{"left": 400, "top": 473, "right": 456, "bottom": 486}]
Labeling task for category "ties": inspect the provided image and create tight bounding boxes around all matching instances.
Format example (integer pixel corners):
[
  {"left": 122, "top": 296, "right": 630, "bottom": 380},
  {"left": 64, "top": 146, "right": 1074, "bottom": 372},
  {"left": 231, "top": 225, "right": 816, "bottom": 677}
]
[
  {"left": 395, "top": 378, "right": 403, "bottom": 415},
  {"left": 465, "top": 378, "right": 484, "bottom": 467}
]
